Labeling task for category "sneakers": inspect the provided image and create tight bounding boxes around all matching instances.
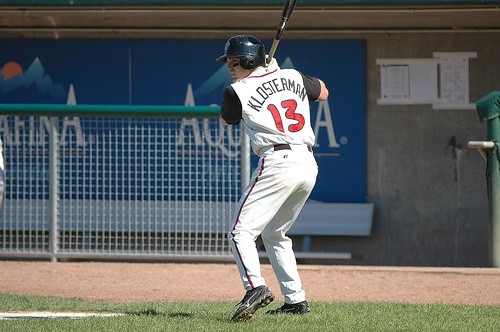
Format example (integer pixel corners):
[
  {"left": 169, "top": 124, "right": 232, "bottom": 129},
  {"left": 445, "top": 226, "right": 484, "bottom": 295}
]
[
  {"left": 265, "top": 300, "right": 310, "bottom": 314},
  {"left": 230, "top": 285, "right": 275, "bottom": 324}
]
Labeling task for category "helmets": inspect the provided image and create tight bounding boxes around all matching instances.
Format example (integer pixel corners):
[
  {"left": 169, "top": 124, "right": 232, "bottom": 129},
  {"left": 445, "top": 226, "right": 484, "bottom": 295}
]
[{"left": 216, "top": 35, "right": 265, "bottom": 70}]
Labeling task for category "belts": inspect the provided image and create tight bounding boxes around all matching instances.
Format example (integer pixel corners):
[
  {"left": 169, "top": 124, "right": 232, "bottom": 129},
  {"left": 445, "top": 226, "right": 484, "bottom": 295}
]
[{"left": 274, "top": 144, "right": 312, "bottom": 151}]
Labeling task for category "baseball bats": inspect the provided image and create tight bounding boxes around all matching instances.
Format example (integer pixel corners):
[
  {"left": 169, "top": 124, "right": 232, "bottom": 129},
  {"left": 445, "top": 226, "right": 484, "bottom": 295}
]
[{"left": 266, "top": 1, "right": 297, "bottom": 67}]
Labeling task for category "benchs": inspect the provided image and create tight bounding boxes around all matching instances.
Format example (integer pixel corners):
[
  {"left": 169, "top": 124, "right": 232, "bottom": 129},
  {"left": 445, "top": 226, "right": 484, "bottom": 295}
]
[{"left": 0, "top": 198, "right": 373, "bottom": 236}]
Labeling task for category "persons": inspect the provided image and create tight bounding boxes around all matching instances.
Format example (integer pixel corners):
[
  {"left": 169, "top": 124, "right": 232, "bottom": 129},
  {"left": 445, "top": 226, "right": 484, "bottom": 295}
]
[{"left": 217, "top": 35, "right": 329, "bottom": 323}]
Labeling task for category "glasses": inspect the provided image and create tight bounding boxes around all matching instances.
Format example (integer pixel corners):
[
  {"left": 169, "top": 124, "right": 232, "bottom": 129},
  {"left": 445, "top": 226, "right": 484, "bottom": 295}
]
[{"left": 226, "top": 61, "right": 240, "bottom": 69}]
